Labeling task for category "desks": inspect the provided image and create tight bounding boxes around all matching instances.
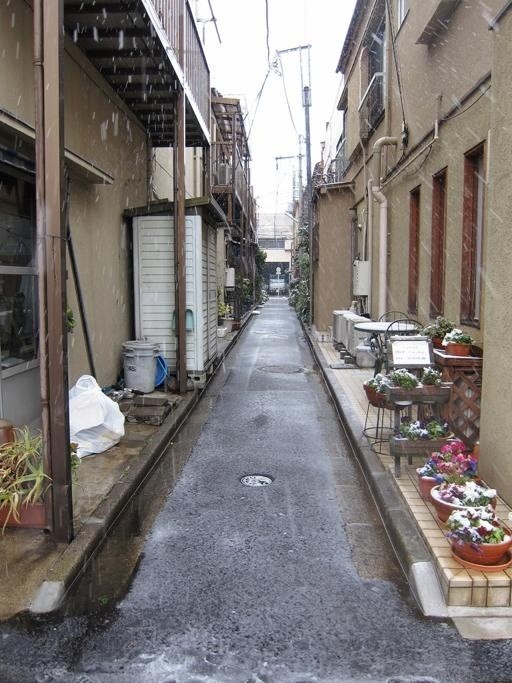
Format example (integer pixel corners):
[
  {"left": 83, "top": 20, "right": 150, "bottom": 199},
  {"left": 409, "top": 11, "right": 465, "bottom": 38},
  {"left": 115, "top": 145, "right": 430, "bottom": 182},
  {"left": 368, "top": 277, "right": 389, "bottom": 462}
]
[{"left": 353, "top": 321, "right": 420, "bottom": 378}]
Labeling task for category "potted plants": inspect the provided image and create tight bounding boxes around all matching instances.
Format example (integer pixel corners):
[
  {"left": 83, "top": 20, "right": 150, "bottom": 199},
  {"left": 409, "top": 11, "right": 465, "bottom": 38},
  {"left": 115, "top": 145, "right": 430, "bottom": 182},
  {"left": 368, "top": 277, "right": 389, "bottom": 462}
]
[
  {"left": 441, "top": 325, "right": 477, "bottom": 356},
  {"left": 362, "top": 366, "right": 454, "bottom": 410},
  {"left": 217, "top": 299, "right": 234, "bottom": 333},
  {"left": 0, "top": 424, "right": 82, "bottom": 529},
  {"left": 413, "top": 315, "right": 457, "bottom": 350}
]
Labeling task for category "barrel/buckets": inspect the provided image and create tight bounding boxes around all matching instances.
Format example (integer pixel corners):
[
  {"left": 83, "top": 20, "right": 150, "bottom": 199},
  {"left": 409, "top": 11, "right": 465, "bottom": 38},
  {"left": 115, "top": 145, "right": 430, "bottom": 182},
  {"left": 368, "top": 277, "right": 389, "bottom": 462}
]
[{"left": 121, "top": 340, "right": 160, "bottom": 393}]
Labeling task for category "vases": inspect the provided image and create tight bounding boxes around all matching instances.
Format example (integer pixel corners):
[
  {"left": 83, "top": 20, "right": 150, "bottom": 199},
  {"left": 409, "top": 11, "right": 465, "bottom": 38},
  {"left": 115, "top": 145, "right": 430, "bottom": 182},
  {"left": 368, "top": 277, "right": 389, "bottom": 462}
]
[
  {"left": 388, "top": 431, "right": 462, "bottom": 478},
  {"left": 429, "top": 484, "right": 497, "bottom": 522},
  {"left": 415, "top": 467, "right": 464, "bottom": 498},
  {"left": 450, "top": 520, "right": 512, "bottom": 565}
]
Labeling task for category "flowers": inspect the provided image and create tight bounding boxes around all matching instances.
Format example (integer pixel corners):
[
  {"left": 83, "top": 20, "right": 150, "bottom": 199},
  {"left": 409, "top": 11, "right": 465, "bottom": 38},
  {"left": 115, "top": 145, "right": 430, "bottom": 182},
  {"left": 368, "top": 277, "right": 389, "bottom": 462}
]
[
  {"left": 390, "top": 415, "right": 451, "bottom": 441},
  {"left": 418, "top": 439, "right": 479, "bottom": 485},
  {"left": 442, "top": 503, "right": 512, "bottom": 556},
  {"left": 438, "top": 465, "right": 497, "bottom": 507}
]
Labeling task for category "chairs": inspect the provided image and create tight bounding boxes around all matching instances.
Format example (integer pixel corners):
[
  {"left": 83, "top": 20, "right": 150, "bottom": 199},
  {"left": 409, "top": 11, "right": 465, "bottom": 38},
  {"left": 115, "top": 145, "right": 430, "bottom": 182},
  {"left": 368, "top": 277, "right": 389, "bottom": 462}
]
[
  {"left": 374, "top": 311, "right": 413, "bottom": 377},
  {"left": 380, "top": 319, "right": 423, "bottom": 375}
]
